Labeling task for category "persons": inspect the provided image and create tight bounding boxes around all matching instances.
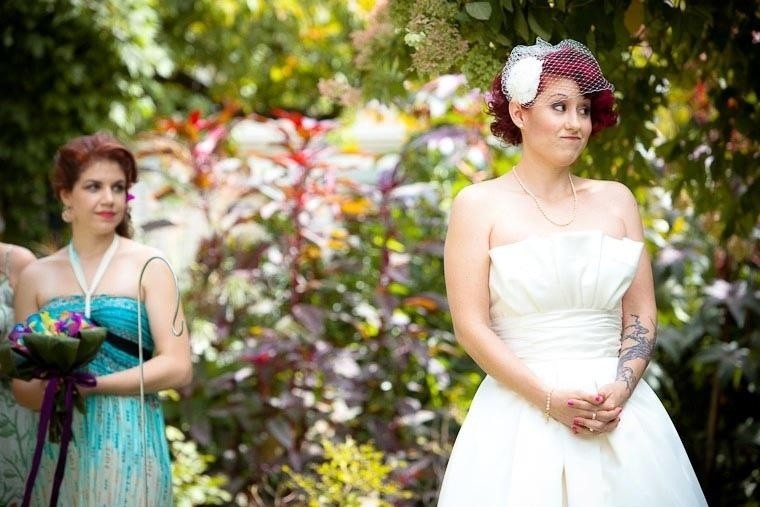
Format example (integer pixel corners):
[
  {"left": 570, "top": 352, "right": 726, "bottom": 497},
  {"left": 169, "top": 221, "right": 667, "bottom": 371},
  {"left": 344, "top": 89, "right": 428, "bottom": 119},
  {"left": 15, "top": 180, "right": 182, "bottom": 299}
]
[
  {"left": 0, "top": 241, "right": 40, "bottom": 507},
  {"left": 8, "top": 133, "right": 196, "bottom": 507},
  {"left": 436, "top": 38, "right": 707, "bottom": 506}
]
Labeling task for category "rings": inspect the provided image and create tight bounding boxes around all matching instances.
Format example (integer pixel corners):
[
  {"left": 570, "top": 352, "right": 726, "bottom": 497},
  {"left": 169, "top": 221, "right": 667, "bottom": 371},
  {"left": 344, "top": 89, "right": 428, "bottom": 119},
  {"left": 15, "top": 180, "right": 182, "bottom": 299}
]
[
  {"left": 589, "top": 428, "right": 594, "bottom": 432},
  {"left": 591, "top": 411, "right": 598, "bottom": 420}
]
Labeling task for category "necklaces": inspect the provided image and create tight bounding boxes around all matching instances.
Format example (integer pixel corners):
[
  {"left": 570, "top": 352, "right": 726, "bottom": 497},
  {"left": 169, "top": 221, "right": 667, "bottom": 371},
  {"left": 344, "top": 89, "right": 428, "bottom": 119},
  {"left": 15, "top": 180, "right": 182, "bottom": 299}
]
[{"left": 510, "top": 162, "right": 580, "bottom": 226}]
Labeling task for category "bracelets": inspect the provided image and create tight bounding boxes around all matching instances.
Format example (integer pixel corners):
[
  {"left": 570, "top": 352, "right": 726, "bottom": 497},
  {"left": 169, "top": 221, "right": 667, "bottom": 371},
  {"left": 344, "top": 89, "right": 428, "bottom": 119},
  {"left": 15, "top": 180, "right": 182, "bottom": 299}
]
[{"left": 543, "top": 390, "right": 553, "bottom": 423}]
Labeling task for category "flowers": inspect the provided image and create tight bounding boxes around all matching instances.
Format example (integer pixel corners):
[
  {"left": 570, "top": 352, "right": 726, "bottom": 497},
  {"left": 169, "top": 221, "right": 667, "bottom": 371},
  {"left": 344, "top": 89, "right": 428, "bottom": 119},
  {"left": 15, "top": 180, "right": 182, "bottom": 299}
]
[{"left": 0, "top": 307, "right": 108, "bottom": 442}]
